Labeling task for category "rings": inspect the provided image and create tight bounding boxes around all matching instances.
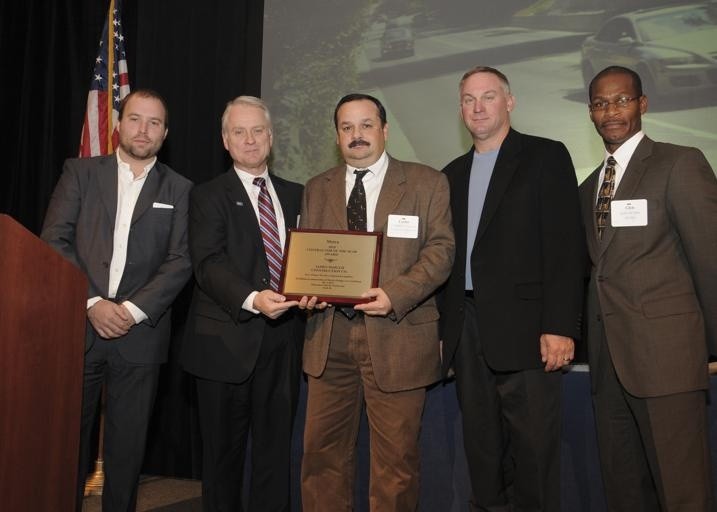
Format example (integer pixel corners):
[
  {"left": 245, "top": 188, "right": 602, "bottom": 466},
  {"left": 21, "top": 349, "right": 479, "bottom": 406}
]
[{"left": 563, "top": 358, "right": 571, "bottom": 361}]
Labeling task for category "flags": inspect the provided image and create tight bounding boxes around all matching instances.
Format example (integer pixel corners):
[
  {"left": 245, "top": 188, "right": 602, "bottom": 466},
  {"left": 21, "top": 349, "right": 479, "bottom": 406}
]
[{"left": 79, "top": 0, "right": 132, "bottom": 158}]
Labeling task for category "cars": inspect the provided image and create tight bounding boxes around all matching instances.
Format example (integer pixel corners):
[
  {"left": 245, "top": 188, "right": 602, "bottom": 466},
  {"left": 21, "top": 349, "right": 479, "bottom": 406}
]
[
  {"left": 583, "top": 3, "right": 717, "bottom": 106},
  {"left": 380, "top": 27, "right": 414, "bottom": 60}
]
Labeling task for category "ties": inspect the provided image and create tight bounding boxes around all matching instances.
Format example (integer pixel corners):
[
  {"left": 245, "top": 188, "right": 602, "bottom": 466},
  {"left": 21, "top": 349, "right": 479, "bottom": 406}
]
[
  {"left": 593, "top": 157, "right": 616, "bottom": 240},
  {"left": 253, "top": 177, "right": 283, "bottom": 293},
  {"left": 340, "top": 170, "right": 369, "bottom": 321}
]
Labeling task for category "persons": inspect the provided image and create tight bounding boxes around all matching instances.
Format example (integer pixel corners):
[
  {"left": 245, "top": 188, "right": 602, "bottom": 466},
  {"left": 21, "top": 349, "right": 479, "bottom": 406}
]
[
  {"left": 176, "top": 94, "right": 306, "bottom": 511},
  {"left": 576, "top": 64, "right": 716, "bottom": 511},
  {"left": 298, "top": 92, "right": 456, "bottom": 512},
  {"left": 435, "top": 65, "right": 587, "bottom": 512},
  {"left": 39, "top": 87, "right": 194, "bottom": 512}
]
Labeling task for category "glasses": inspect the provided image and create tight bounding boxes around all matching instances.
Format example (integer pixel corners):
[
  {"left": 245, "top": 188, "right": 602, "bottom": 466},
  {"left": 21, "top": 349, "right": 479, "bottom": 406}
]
[{"left": 587, "top": 94, "right": 641, "bottom": 111}]
